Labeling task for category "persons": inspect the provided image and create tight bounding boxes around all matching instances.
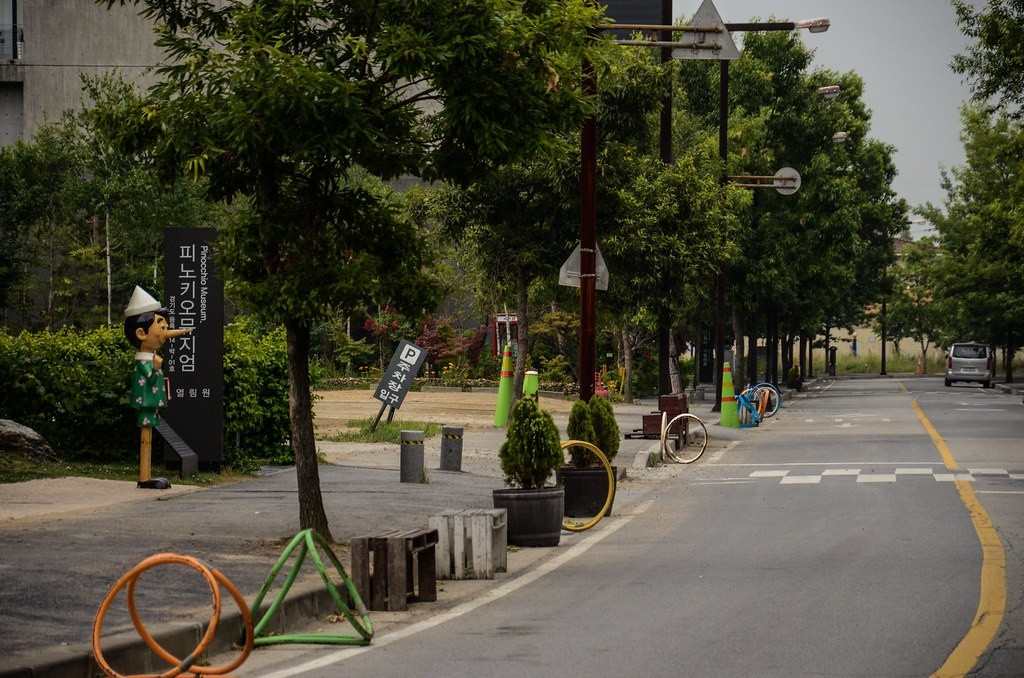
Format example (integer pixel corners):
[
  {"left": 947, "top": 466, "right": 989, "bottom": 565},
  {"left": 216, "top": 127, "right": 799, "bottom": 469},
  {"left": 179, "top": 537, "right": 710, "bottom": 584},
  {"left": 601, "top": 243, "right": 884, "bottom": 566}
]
[{"left": 123, "top": 285, "right": 191, "bottom": 490}]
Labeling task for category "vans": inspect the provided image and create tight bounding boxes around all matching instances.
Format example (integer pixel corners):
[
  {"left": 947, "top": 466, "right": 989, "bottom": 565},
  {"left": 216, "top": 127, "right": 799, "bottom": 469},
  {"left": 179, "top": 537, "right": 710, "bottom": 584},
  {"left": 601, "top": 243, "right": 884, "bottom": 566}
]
[{"left": 944, "top": 342, "right": 993, "bottom": 389}]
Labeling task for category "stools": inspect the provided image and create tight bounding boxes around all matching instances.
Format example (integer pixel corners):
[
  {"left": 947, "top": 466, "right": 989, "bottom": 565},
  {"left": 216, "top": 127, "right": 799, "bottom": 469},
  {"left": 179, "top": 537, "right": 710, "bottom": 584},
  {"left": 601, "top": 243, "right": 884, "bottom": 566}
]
[
  {"left": 428, "top": 507, "right": 508, "bottom": 579},
  {"left": 351, "top": 527, "right": 439, "bottom": 611}
]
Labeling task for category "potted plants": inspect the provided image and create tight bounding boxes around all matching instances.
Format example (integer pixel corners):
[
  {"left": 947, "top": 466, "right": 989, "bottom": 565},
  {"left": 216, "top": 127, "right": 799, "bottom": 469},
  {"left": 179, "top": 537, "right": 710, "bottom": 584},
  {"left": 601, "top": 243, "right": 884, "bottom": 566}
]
[
  {"left": 786, "top": 367, "right": 802, "bottom": 392},
  {"left": 555, "top": 395, "right": 621, "bottom": 517},
  {"left": 492, "top": 408, "right": 566, "bottom": 547}
]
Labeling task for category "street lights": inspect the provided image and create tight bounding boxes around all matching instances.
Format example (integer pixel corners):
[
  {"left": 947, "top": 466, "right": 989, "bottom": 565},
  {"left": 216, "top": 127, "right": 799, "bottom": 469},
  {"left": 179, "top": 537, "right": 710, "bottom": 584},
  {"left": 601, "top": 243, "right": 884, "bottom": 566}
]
[
  {"left": 708, "top": 16, "right": 833, "bottom": 412},
  {"left": 769, "top": 131, "right": 849, "bottom": 394},
  {"left": 765, "top": 85, "right": 841, "bottom": 413}
]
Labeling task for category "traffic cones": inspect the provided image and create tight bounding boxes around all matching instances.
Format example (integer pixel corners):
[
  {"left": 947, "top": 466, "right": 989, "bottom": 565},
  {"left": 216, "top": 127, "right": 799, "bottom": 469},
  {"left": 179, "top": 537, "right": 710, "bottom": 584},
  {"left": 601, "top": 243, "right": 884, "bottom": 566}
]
[
  {"left": 721, "top": 362, "right": 739, "bottom": 427},
  {"left": 494, "top": 345, "right": 513, "bottom": 427}
]
